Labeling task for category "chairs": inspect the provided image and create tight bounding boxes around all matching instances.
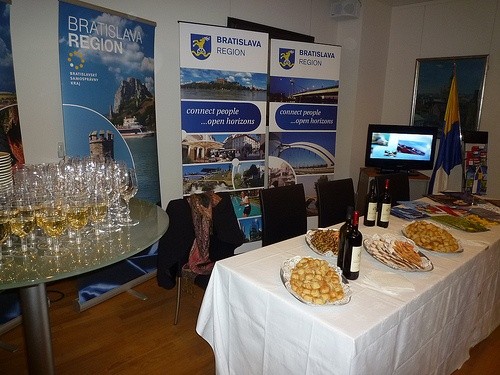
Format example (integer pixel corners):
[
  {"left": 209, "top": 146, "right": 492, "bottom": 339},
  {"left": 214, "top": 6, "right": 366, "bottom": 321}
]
[
  {"left": 154, "top": 191, "right": 249, "bottom": 326},
  {"left": 373, "top": 168, "right": 410, "bottom": 209},
  {"left": 316, "top": 177, "right": 355, "bottom": 228},
  {"left": 259, "top": 184, "right": 307, "bottom": 245}
]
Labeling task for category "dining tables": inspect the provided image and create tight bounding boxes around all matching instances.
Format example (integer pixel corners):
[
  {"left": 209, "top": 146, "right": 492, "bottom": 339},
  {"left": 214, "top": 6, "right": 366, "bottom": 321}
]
[{"left": 214, "top": 190, "right": 500, "bottom": 375}]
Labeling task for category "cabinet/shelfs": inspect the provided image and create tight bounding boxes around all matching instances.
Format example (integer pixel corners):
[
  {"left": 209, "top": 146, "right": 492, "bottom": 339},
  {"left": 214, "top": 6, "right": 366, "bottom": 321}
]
[{"left": 361, "top": 167, "right": 430, "bottom": 204}]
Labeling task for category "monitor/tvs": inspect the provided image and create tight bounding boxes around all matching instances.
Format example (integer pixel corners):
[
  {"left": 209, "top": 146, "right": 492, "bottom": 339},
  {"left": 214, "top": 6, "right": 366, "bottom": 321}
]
[{"left": 363, "top": 125, "right": 438, "bottom": 175}]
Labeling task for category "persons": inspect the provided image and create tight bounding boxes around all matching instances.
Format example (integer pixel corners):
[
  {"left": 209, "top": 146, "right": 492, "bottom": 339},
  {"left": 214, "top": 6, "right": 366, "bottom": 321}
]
[{"left": 241, "top": 191, "right": 252, "bottom": 218}]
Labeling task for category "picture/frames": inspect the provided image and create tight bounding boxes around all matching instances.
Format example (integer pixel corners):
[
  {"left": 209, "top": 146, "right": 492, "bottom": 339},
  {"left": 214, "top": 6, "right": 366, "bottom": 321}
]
[{"left": 408, "top": 54, "right": 489, "bottom": 131}]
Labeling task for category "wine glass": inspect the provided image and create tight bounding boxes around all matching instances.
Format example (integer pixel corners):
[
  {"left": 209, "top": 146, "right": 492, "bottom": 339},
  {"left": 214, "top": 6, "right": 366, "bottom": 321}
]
[{"left": 0, "top": 152, "right": 140, "bottom": 265}]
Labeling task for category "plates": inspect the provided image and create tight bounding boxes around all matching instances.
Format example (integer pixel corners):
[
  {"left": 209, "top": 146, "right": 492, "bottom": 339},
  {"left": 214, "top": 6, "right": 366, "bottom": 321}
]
[
  {"left": 363, "top": 238, "right": 433, "bottom": 272},
  {"left": 305, "top": 228, "right": 340, "bottom": 257},
  {"left": 280, "top": 268, "right": 351, "bottom": 304}
]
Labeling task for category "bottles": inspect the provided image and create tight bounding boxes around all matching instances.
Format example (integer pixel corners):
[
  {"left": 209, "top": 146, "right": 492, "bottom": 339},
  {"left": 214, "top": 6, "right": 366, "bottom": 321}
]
[
  {"left": 336, "top": 207, "right": 354, "bottom": 269},
  {"left": 364, "top": 179, "right": 377, "bottom": 227},
  {"left": 377, "top": 179, "right": 391, "bottom": 228},
  {"left": 342, "top": 210, "right": 363, "bottom": 280}
]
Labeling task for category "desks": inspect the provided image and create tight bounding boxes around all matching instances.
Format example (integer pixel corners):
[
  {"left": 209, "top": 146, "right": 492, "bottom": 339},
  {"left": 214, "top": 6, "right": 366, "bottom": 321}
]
[{"left": 0, "top": 198, "right": 172, "bottom": 375}]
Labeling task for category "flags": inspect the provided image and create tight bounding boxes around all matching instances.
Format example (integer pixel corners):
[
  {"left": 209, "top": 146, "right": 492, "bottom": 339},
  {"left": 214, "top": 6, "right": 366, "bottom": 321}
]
[{"left": 428, "top": 64, "right": 467, "bottom": 194}]
